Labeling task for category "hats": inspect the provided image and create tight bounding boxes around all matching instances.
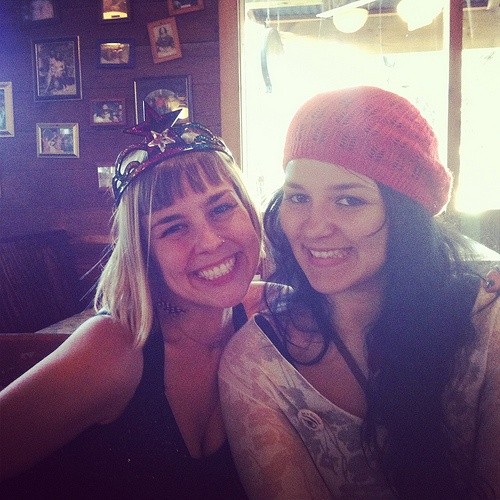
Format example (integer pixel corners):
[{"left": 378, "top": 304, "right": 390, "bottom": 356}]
[{"left": 283, "top": 85, "right": 452, "bottom": 216}]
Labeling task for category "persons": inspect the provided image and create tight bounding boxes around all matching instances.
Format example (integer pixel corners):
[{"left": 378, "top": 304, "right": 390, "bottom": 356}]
[
  {"left": 0, "top": 124, "right": 263, "bottom": 500},
  {"left": 155, "top": 27, "right": 176, "bottom": 54},
  {"left": 215, "top": 88, "right": 500, "bottom": 500}
]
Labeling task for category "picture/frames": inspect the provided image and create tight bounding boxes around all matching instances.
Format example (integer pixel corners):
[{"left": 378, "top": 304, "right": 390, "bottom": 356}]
[
  {"left": 97, "top": 0, "right": 133, "bottom": 24},
  {"left": 35, "top": 121, "right": 81, "bottom": 160},
  {"left": 31, "top": 35, "right": 83, "bottom": 104},
  {"left": 95, "top": 162, "right": 116, "bottom": 193},
  {"left": 28, "top": 0, "right": 58, "bottom": 25},
  {"left": 88, "top": 96, "right": 128, "bottom": 128},
  {"left": 0, "top": 81, "right": 15, "bottom": 139},
  {"left": 95, "top": 37, "right": 137, "bottom": 69},
  {"left": 167, "top": 0, "right": 206, "bottom": 17},
  {"left": 132, "top": 74, "right": 195, "bottom": 137},
  {"left": 146, "top": 16, "right": 184, "bottom": 65}
]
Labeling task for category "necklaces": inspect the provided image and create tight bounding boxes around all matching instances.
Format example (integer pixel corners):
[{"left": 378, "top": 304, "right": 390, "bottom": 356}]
[{"left": 157, "top": 295, "right": 230, "bottom": 352}]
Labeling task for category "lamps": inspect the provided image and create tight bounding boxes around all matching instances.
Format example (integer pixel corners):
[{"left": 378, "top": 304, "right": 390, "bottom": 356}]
[
  {"left": 396, "top": 0, "right": 444, "bottom": 30},
  {"left": 332, "top": 6, "right": 369, "bottom": 35}
]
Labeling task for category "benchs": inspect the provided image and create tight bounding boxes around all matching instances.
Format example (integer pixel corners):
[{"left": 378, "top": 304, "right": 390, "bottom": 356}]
[{"left": 0, "top": 228, "right": 81, "bottom": 395}]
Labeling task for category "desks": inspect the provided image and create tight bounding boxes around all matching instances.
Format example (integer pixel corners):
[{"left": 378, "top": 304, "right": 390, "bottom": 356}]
[{"left": 34, "top": 303, "right": 101, "bottom": 335}]
